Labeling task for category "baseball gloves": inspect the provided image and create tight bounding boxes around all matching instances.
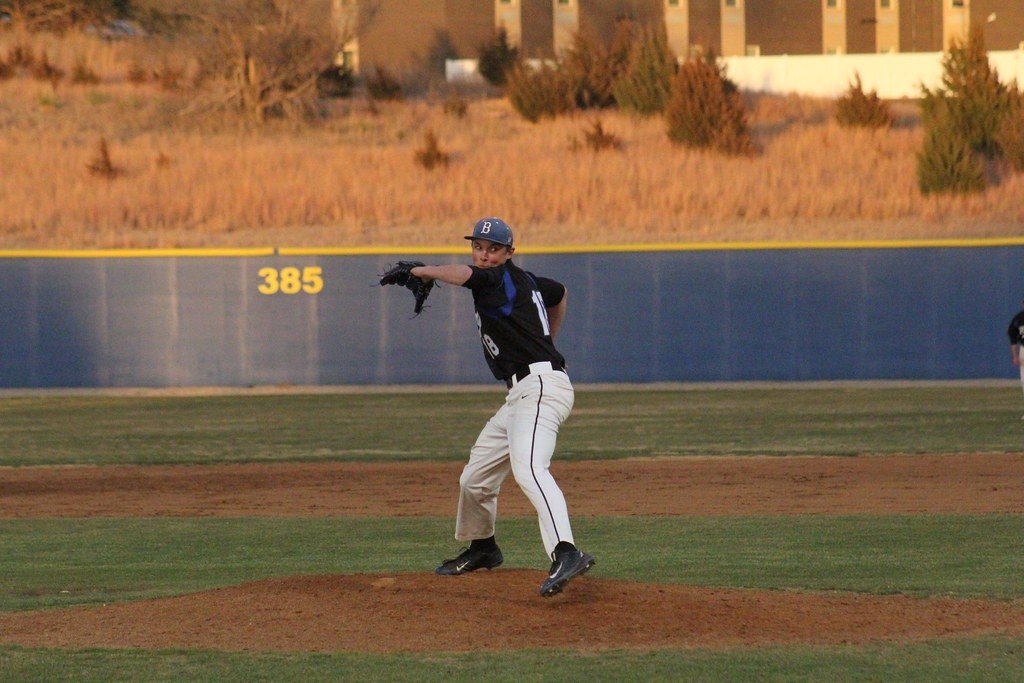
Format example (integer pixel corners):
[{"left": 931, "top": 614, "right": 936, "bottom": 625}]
[{"left": 380, "top": 261, "right": 435, "bottom": 313}]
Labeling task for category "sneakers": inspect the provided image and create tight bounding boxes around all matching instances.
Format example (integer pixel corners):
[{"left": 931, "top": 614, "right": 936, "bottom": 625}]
[
  {"left": 540, "top": 549, "right": 596, "bottom": 598},
  {"left": 436, "top": 545, "right": 503, "bottom": 575}
]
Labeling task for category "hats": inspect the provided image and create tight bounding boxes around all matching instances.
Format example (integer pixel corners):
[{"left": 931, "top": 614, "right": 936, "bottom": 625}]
[{"left": 464, "top": 217, "right": 513, "bottom": 247}]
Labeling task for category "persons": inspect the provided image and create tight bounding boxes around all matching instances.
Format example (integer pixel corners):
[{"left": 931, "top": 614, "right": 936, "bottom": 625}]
[
  {"left": 1007, "top": 311, "right": 1024, "bottom": 391},
  {"left": 380, "top": 217, "right": 596, "bottom": 597}
]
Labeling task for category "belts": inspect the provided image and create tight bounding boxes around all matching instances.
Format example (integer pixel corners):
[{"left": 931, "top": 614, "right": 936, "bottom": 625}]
[{"left": 507, "top": 367, "right": 565, "bottom": 390}]
